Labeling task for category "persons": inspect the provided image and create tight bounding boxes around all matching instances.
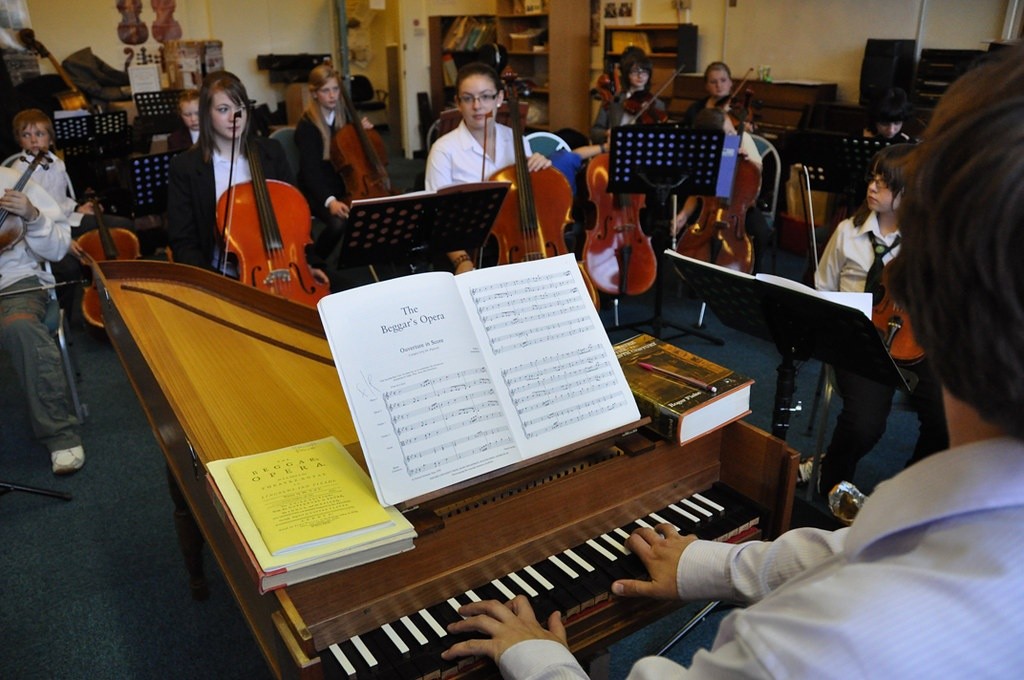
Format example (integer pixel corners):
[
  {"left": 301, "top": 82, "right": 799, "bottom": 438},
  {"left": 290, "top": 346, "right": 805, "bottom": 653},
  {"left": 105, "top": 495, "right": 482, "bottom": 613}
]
[
  {"left": 165, "top": 69, "right": 341, "bottom": 293},
  {"left": 293, "top": 65, "right": 374, "bottom": 279},
  {"left": 166, "top": 88, "right": 200, "bottom": 150},
  {"left": 796, "top": 142, "right": 949, "bottom": 502},
  {"left": 442, "top": 38, "right": 1024, "bottom": 679},
  {"left": 0, "top": 165, "right": 88, "bottom": 476},
  {"left": 11, "top": 109, "right": 137, "bottom": 324},
  {"left": 842, "top": 85, "right": 920, "bottom": 218},
  {"left": 551, "top": 45, "right": 765, "bottom": 251},
  {"left": 425, "top": 62, "right": 553, "bottom": 275}
]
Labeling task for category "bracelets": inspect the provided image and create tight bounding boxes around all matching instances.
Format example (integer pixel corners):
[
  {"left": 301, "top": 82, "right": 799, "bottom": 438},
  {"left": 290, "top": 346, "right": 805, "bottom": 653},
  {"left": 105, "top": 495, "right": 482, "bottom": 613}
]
[{"left": 453, "top": 255, "right": 472, "bottom": 268}]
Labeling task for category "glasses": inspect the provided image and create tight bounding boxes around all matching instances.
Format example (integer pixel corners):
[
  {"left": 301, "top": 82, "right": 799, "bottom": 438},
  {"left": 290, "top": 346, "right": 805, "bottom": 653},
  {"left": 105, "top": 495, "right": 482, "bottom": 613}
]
[
  {"left": 864, "top": 171, "right": 889, "bottom": 189},
  {"left": 630, "top": 68, "right": 651, "bottom": 77},
  {"left": 458, "top": 90, "right": 500, "bottom": 105}
]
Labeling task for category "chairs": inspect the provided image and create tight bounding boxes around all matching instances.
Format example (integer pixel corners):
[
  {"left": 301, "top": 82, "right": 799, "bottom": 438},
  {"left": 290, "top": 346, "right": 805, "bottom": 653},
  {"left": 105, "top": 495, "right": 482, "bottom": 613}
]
[
  {"left": 351, "top": 75, "right": 388, "bottom": 114},
  {"left": 42, "top": 261, "right": 87, "bottom": 424},
  {"left": 805, "top": 363, "right": 907, "bottom": 502},
  {"left": 751, "top": 134, "right": 780, "bottom": 274}
]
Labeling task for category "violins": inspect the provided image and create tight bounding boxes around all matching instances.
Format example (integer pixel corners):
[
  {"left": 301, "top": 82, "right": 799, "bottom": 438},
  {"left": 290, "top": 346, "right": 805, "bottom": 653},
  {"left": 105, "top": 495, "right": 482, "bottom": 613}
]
[
  {"left": 870, "top": 254, "right": 929, "bottom": 368},
  {"left": 706, "top": 94, "right": 755, "bottom": 131},
  {"left": 624, "top": 90, "right": 668, "bottom": 124},
  {"left": 0, "top": 146, "right": 47, "bottom": 279}
]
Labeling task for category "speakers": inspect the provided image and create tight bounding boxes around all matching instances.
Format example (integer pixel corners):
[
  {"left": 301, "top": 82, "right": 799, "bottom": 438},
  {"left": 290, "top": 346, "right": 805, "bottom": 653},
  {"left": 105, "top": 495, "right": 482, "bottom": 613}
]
[{"left": 858, "top": 40, "right": 916, "bottom": 108}]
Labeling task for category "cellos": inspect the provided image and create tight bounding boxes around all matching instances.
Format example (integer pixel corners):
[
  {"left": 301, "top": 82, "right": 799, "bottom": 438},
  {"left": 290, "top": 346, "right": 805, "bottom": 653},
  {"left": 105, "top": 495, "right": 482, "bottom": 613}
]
[
  {"left": 17, "top": 26, "right": 91, "bottom": 111},
  {"left": 675, "top": 88, "right": 762, "bottom": 276},
  {"left": 581, "top": 74, "right": 657, "bottom": 295},
  {"left": 78, "top": 193, "right": 144, "bottom": 332},
  {"left": 486, "top": 64, "right": 599, "bottom": 313},
  {"left": 216, "top": 96, "right": 330, "bottom": 310},
  {"left": 331, "top": 70, "right": 401, "bottom": 207}
]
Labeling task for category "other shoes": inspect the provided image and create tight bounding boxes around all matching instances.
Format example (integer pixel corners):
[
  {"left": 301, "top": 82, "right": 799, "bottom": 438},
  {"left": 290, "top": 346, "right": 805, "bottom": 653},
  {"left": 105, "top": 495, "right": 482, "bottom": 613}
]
[
  {"left": 50, "top": 444, "right": 85, "bottom": 473},
  {"left": 796, "top": 455, "right": 815, "bottom": 484}
]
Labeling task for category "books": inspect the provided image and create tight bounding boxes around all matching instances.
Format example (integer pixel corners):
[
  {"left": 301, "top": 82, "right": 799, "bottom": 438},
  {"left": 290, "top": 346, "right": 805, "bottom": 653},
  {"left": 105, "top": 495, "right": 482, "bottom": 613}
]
[
  {"left": 612, "top": 332, "right": 756, "bottom": 447},
  {"left": 315, "top": 250, "right": 642, "bottom": 507},
  {"left": 343, "top": 182, "right": 513, "bottom": 268},
  {"left": 441, "top": 17, "right": 497, "bottom": 52},
  {"left": 205, "top": 435, "right": 418, "bottom": 596},
  {"left": 440, "top": 54, "right": 459, "bottom": 87}
]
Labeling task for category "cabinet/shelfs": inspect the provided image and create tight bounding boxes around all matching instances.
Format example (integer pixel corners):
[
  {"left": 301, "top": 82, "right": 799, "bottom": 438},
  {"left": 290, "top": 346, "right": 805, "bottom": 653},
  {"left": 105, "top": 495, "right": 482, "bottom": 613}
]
[
  {"left": 429, "top": 0, "right": 590, "bottom": 138},
  {"left": 603, "top": 22, "right": 698, "bottom": 98}
]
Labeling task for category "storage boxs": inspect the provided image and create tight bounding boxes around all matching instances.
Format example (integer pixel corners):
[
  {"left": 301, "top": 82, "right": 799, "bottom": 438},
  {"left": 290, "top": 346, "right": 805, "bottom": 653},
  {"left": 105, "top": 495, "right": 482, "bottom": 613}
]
[{"left": 512, "top": 35, "right": 543, "bottom": 51}]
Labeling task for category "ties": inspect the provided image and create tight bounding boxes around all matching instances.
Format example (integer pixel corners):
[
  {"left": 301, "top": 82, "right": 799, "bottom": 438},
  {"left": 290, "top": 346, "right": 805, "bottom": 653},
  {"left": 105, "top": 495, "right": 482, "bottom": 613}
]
[{"left": 864, "top": 230, "right": 901, "bottom": 306}]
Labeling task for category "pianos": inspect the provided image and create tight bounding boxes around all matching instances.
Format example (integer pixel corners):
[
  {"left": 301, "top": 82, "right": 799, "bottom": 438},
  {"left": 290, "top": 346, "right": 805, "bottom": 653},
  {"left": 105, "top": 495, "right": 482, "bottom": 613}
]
[{"left": 63, "top": 258, "right": 802, "bottom": 680}]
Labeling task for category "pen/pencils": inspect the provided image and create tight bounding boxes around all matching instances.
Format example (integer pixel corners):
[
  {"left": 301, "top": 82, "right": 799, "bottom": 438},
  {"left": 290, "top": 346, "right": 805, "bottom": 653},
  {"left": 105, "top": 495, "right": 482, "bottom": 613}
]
[{"left": 635, "top": 361, "right": 720, "bottom": 392}]
